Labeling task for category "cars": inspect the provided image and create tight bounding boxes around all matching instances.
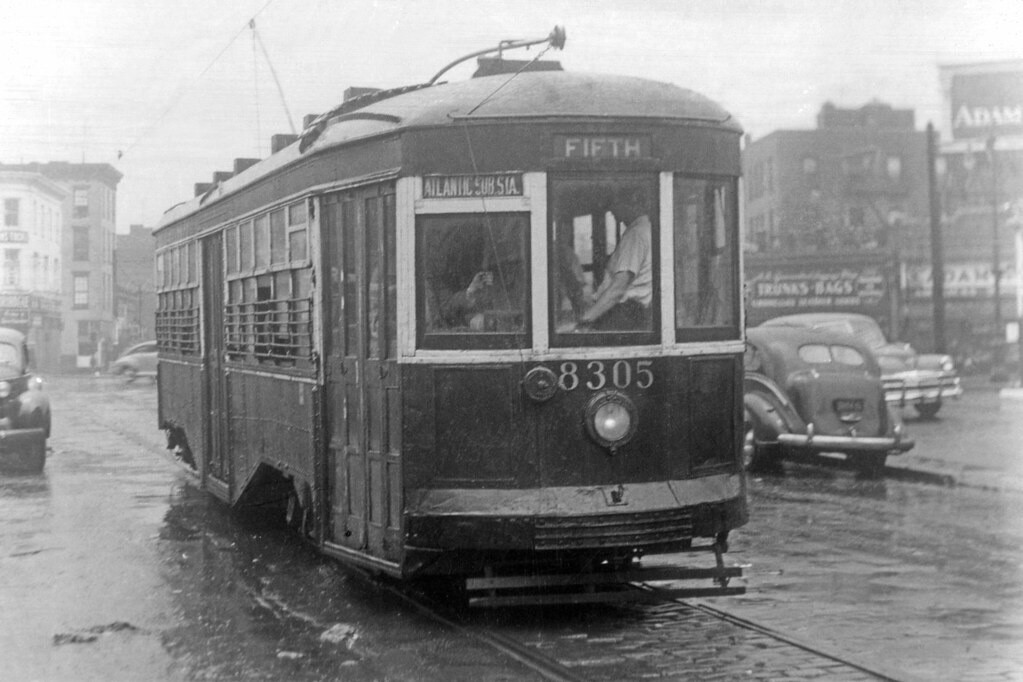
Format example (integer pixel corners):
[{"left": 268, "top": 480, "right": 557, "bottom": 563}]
[
  {"left": 760, "top": 311, "right": 961, "bottom": 420},
  {"left": 740, "top": 325, "right": 915, "bottom": 474},
  {"left": 109, "top": 341, "right": 158, "bottom": 385},
  {"left": 0, "top": 327, "right": 55, "bottom": 476}
]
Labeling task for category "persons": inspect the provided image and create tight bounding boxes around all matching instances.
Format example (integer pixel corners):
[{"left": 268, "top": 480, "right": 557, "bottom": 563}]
[{"left": 426, "top": 181, "right": 654, "bottom": 332}]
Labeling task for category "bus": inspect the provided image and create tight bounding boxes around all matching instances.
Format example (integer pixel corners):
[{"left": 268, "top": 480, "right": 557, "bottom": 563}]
[{"left": 150, "top": 26, "right": 750, "bottom": 612}]
